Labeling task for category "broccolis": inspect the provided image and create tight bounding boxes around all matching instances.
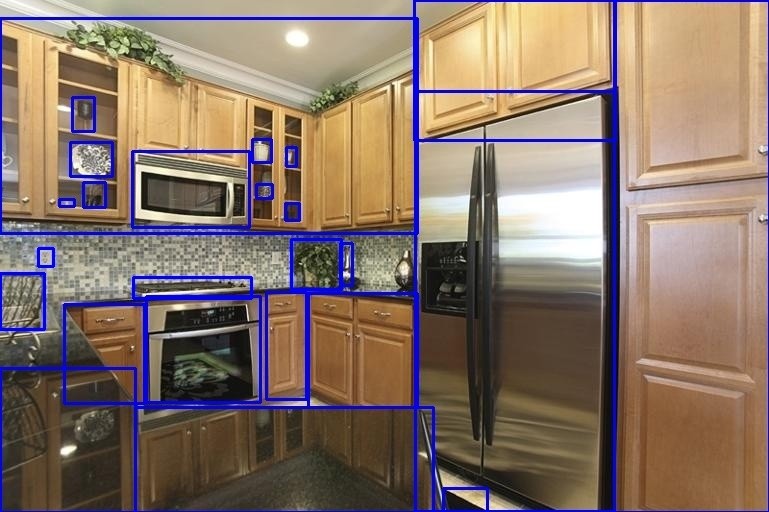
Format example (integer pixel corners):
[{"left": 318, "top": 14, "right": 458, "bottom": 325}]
[{"left": 415, "top": 92, "right": 614, "bottom": 512}]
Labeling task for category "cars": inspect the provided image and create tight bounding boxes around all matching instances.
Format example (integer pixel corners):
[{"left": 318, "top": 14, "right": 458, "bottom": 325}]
[
  {"left": 253, "top": 140, "right": 272, "bottom": 163},
  {"left": 257, "top": 184, "right": 272, "bottom": 198},
  {"left": 83, "top": 183, "right": 105, "bottom": 208},
  {"left": 285, "top": 203, "right": 299, "bottom": 220},
  {"left": 286, "top": 148, "right": 297, "bottom": 166},
  {"left": 60, "top": 199, "right": 74, "bottom": 207}
]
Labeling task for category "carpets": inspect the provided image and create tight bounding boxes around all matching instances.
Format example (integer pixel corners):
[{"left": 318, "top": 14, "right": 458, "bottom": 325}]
[{"left": 444, "top": 489, "right": 488, "bottom": 512}]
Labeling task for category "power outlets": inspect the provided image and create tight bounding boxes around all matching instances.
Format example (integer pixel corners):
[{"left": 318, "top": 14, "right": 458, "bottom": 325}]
[{"left": 38, "top": 249, "right": 53, "bottom": 267}]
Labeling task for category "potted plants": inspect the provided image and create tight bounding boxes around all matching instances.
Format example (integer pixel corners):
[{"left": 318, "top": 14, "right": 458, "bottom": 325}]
[{"left": 291, "top": 239, "right": 341, "bottom": 289}]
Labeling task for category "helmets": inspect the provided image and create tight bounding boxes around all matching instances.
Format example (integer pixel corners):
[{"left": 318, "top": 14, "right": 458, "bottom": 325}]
[{"left": 71, "top": 142, "right": 112, "bottom": 177}]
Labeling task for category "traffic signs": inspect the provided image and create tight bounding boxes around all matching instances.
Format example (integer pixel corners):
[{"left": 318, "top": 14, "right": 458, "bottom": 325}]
[{"left": 38, "top": 249, "right": 53, "bottom": 267}]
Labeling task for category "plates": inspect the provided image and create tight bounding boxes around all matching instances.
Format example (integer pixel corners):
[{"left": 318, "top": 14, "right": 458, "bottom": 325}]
[
  {"left": 64, "top": 304, "right": 144, "bottom": 403},
  {"left": 0, "top": 369, "right": 135, "bottom": 512},
  {"left": 135, "top": 407, "right": 433, "bottom": 512},
  {"left": 614, "top": 0, "right": 769, "bottom": 512},
  {"left": 415, "top": 0, "right": 616, "bottom": 140},
  {"left": 309, "top": 294, "right": 418, "bottom": 407},
  {"left": 266, "top": 293, "right": 307, "bottom": 400}
]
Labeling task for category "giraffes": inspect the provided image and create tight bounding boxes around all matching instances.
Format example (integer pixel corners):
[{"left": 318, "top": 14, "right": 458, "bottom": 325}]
[{"left": 133, "top": 278, "right": 251, "bottom": 299}]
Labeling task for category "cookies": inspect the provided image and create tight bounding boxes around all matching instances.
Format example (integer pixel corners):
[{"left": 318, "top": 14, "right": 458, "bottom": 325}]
[{"left": 132, "top": 152, "right": 249, "bottom": 228}]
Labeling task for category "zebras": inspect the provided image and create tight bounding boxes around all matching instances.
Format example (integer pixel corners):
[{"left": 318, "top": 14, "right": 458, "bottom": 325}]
[{"left": 146, "top": 296, "right": 260, "bottom": 403}]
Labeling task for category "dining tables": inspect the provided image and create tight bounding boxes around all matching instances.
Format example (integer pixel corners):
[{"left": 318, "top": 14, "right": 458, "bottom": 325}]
[{"left": 291, "top": 239, "right": 341, "bottom": 289}]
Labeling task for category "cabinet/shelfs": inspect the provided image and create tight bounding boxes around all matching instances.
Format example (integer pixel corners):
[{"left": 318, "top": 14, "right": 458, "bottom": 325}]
[
  {"left": 250, "top": 99, "right": 314, "bottom": 230},
  {"left": 0, "top": 19, "right": 130, "bottom": 219},
  {"left": 135, "top": 407, "right": 433, "bottom": 512},
  {"left": 266, "top": 293, "right": 307, "bottom": 400},
  {"left": 341, "top": 243, "right": 353, "bottom": 285},
  {"left": 316, "top": 97, "right": 352, "bottom": 226},
  {"left": 64, "top": 304, "right": 144, "bottom": 403},
  {"left": 135, "top": 60, "right": 249, "bottom": 170},
  {"left": 614, "top": 0, "right": 769, "bottom": 512},
  {"left": 73, "top": 97, "right": 95, "bottom": 131},
  {"left": 0, "top": 369, "right": 135, "bottom": 512},
  {"left": 352, "top": 70, "right": 414, "bottom": 226},
  {"left": 415, "top": 0, "right": 616, "bottom": 140},
  {"left": 309, "top": 294, "right": 418, "bottom": 407}
]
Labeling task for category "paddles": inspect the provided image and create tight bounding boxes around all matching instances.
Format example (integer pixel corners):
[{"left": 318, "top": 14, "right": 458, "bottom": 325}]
[{"left": 444, "top": 489, "right": 488, "bottom": 512}]
